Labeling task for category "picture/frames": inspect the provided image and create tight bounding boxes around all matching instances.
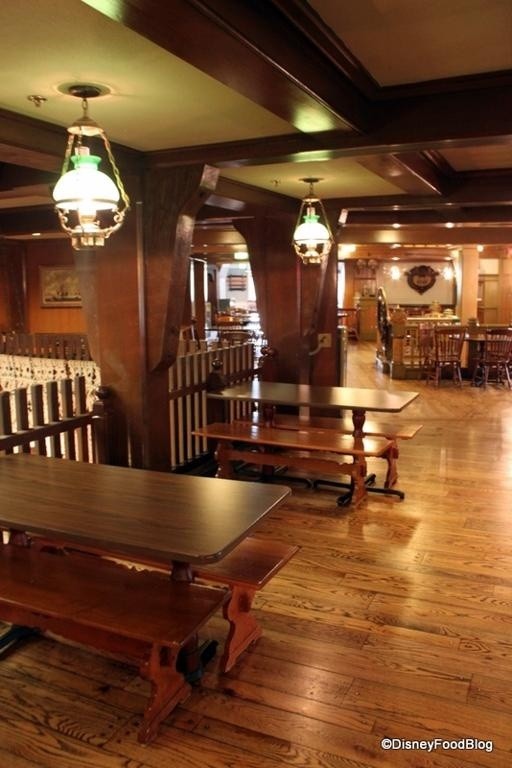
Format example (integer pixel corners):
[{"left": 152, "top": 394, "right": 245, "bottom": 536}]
[{"left": 37, "top": 265, "right": 84, "bottom": 308}]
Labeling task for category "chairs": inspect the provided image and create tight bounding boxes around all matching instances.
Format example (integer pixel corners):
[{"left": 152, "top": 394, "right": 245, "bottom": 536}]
[
  {"left": 482, "top": 327, "right": 512, "bottom": 391},
  {"left": 424, "top": 326, "right": 467, "bottom": 390}
]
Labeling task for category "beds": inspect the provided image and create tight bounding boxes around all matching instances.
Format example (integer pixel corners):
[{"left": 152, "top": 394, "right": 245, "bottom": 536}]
[{"left": 3, "top": 454, "right": 291, "bottom": 701}]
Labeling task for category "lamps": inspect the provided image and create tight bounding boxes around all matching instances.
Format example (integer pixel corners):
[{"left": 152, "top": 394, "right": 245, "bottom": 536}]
[
  {"left": 290, "top": 178, "right": 334, "bottom": 266},
  {"left": 51, "top": 84, "right": 134, "bottom": 248}
]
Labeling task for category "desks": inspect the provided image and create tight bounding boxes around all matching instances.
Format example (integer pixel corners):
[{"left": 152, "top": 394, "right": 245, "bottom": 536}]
[
  {"left": 448, "top": 334, "right": 512, "bottom": 388},
  {"left": 400, "top": 314, "right": 462, "bottom": 367},
  {"left": 207, "top": 380, "right": 420, "bottom": 505}
]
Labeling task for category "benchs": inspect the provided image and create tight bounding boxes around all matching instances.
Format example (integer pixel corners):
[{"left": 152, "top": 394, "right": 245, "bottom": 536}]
[
  {"left": 12, "top": 531, "right": 304, "bottom": 679},
  {"left": 235, "top": 413, "right": 425, "bottom": 495},
  {"left": 190, "top": 422, "right": 396, "bottom": 510},
  {"left": 0, "top": 543, "right": 235, "bottom": 746}
]
